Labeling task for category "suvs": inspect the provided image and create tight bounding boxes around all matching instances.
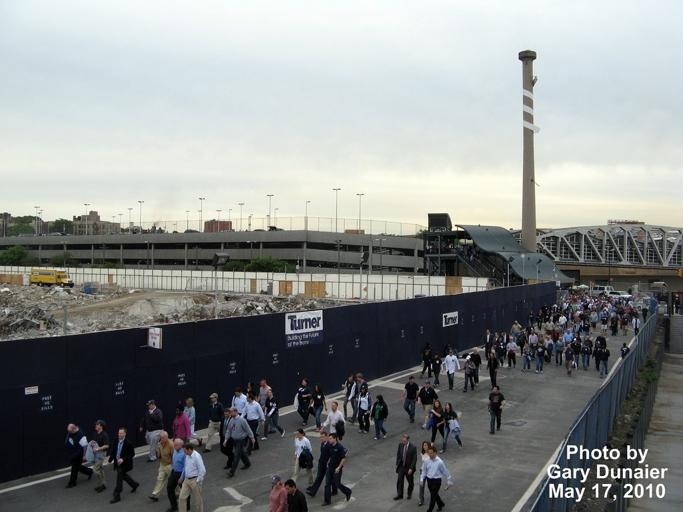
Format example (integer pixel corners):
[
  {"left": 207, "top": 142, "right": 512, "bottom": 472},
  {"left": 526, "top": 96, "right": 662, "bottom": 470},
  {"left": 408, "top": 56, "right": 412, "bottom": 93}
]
[{"left": 608, "top": 290, "right": 633, "bottom": 298}]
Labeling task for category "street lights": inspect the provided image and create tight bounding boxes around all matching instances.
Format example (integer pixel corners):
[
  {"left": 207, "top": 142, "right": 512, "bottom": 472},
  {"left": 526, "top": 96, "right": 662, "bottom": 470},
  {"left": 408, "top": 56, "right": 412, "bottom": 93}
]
[{"left": 0, "top": 187, "right": 374, "bottom": 280}]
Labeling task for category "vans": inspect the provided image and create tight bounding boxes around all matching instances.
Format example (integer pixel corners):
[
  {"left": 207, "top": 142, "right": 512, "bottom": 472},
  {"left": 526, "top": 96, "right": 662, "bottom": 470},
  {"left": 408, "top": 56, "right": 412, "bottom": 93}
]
[{"left": 587, "top": 286, "right": 613, "bottom": 297}]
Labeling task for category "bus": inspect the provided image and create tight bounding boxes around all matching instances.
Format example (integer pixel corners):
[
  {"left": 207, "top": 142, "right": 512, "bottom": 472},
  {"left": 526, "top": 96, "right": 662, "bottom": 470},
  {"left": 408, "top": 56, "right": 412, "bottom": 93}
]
[{"left": 27, "top": 266, "right": 77, "bottom": 289}]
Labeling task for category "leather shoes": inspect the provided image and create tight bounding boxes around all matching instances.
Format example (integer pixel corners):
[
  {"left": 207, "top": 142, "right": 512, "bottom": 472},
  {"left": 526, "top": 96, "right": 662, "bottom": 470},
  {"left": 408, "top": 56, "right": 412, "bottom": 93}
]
[
  {"left": 131, "top": 483, "right": 139, "bottom": 492},
  {"left": 110, "top": 497, "right": 120, "bottom": 503}
]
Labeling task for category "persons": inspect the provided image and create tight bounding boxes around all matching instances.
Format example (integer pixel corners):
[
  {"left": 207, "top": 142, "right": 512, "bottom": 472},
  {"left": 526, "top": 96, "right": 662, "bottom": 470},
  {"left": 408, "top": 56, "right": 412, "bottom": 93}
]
[{"left": 65, "top": 292, "right": 682, "bottom": 510}]
